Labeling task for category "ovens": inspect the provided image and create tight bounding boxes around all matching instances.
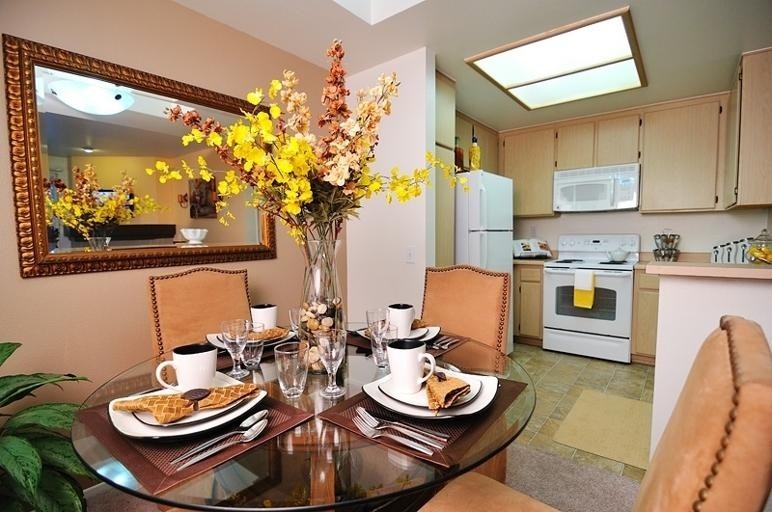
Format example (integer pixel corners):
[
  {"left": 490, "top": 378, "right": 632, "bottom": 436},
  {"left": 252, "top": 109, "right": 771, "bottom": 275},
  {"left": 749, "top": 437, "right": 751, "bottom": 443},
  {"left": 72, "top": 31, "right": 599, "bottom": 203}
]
[{"left": 542, "top": 268, "right": 635, "bottom": 365}]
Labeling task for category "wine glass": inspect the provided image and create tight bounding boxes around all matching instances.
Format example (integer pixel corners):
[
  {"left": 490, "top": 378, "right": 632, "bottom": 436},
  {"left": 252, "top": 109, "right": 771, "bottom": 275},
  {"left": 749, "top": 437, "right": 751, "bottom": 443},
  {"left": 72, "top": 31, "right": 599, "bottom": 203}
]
[
  {"left": 363, "top": 311, "right": 389, "bottom": 333},
  {"left": 314, "top": 328, "right": 348, "bottom": 402},
  {"left": 219, "top": 320, "right": 251, "bottom": 375}
]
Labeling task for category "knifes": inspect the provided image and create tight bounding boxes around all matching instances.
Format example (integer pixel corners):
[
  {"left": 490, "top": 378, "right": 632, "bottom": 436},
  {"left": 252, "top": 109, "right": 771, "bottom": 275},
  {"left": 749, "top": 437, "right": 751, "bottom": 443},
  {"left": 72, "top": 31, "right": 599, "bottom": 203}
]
[{"left": 355, "top": 406, "right": 452, "bottom": 442}]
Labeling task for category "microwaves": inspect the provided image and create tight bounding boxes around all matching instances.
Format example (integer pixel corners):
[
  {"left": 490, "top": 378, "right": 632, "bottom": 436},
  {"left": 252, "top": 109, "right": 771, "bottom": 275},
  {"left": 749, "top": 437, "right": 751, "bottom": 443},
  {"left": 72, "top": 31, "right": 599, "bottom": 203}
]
[{"left": 551, "top": 162, "right": 640, "bottom": 215}]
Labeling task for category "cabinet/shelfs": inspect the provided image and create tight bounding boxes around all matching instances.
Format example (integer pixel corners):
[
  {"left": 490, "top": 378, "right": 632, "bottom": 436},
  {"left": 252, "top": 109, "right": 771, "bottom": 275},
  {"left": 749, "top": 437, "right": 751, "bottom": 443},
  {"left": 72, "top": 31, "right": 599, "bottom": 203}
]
[
  {"left": 641, "top": 101, "right": 722, "bottom": 210},
  {"left": 723, "top": 55, "right": 767, "bottom": 209},
  {"left": 517, "top": 264, "right": 542, "bottom": 340},
  {"left": 555, "top": 115, "right": 642, "bottom": 171},
  {"left": 632, "top": 270, "right": 659, "bottom": 357},
  {"left": 502, "top": 128, "right": 555, "bottom": 217}
]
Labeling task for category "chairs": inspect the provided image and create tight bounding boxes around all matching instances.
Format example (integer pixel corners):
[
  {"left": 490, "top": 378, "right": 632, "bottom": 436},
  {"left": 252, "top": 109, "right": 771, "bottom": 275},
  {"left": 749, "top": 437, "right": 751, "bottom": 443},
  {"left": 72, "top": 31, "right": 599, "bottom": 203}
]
[
  {"left": 416, "top": 315, "right": 772, "bottom": 511},
  {"left": 147, "top": 267, "right": 253, "bottom": 388},
  {"left": 420, "top": 264, "right": 511, "bottom": 374}
]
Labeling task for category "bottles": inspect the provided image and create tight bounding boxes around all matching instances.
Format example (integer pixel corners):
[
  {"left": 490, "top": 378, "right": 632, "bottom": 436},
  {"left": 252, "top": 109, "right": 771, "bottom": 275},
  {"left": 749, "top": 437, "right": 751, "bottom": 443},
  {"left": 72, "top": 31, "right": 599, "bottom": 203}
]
[
  {"left": 454, "top": 137, "right": 464, "bottom": 172},
  {"left": 293, "top": 239, "right": 353, "bottom": 375},
  {"left": 468, "top": 137, "right": 480, "bottom": 170}
]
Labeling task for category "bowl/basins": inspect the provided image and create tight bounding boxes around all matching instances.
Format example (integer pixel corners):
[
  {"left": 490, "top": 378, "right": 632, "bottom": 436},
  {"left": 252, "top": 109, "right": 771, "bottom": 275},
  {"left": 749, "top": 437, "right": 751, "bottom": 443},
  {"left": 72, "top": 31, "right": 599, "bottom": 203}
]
[{"left": 180, "top": 226, "right": 210, "bottom": 246}]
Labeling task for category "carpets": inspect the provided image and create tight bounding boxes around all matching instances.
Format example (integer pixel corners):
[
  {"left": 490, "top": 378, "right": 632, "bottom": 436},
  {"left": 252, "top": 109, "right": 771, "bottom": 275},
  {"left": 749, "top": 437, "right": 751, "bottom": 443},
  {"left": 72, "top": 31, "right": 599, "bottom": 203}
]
[{"left": 553, "top": 390, "right": 651, "bottom": 471}]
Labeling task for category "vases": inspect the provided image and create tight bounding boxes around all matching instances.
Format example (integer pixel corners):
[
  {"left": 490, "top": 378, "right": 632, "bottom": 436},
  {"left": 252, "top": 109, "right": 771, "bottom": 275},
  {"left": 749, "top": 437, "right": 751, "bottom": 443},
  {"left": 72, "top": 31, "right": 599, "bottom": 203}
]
[
  {"left": 295, "top": 242, "right": 347, "bottom": 373},
  {"left": 86, "top": 236, "right": 111, "bottom": 249}
]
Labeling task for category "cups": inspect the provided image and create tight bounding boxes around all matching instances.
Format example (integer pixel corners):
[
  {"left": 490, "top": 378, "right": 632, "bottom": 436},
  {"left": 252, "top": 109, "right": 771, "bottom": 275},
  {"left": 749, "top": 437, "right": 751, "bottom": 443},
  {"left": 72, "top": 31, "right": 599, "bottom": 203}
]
[
  {"left": 155, "top": 343, "right": 219, "bottom": 390},
  {"left": 272, "top": 343, "right": 312, "bottom": 401},
  {"left": 243, "top": 336, "right": 265, "bottom": 374},
  {"left": 387, "top": 339, "right": 431, "bottom": 394},
  {"left": 388, "top": 301, "right": 418, "bottom": 335},
  {"left": 368, "top": 336, "right": 393, "bottom": 372},
  {"left": 251, "top": 303, "right": 281, "bottom": 329}
]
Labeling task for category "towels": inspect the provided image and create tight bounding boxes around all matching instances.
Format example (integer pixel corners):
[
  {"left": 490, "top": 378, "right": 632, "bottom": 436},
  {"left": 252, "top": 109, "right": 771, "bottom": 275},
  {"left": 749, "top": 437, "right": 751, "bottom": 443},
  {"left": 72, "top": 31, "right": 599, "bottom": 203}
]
[
  {"left": 248, "top": 326, "right": 289, "bottom": 341},
  {"left": 112, "top": 383, "right": 259, "bottom": 424},
  {"left": 364, "top": 318, "right": 423, "bottom": 337},
  {"left": 424, "top": 367, "right": 470, "bottom": 419}
]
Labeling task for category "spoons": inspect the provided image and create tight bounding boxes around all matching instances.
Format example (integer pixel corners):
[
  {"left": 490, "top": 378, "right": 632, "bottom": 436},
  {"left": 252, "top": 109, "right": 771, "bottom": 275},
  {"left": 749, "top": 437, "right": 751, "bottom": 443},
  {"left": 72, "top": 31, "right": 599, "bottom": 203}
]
[
  {"left": 174, "top": 418, "right": 269, "bottom": 471},
  {"left": 167, "top": 409, "right": 271, "bottom": 464}
]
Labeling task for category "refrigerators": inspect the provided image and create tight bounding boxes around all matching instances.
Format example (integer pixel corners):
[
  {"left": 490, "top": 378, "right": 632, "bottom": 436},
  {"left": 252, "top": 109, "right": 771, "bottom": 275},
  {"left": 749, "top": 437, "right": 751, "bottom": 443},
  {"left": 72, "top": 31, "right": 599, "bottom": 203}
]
[{"left": 452, "top": 170, "right": 519, "bottom": 356}]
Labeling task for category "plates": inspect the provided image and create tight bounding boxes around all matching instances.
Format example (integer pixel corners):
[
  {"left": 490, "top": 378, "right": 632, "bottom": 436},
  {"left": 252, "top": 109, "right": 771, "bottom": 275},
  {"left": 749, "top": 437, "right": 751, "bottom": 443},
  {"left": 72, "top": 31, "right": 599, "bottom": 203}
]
[
  {"left": 208, "top": 329, "right": 299, "bottom": 344},
  {"left": 356, "top": 327, "right": 446, "bottom": 340},
  {"left": 363, "top": 362, "right": 502, "bottom": 418},
  {"left": 109, "top": 373, "right": 268, "bottom": 445}
]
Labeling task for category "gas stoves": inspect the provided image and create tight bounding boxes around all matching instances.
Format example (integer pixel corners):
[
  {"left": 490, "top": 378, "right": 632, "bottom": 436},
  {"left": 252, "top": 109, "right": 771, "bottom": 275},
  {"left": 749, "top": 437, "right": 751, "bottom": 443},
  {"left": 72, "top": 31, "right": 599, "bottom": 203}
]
[{"left": 545, "top": 256, "right": 637, "bottom": 274}]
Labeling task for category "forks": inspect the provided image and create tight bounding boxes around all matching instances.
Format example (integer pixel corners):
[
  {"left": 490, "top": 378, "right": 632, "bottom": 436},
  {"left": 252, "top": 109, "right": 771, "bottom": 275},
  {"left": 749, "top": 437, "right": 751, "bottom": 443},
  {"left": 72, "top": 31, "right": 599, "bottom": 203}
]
[
  {"left": 430, "top": 335, "right": 460, "bottom": 352},
  {"left": 347, "top": 415, "right": 433, "bottom": 458},
  {"left": 354, "top": 404, "right": 444, "bottom": 450}
]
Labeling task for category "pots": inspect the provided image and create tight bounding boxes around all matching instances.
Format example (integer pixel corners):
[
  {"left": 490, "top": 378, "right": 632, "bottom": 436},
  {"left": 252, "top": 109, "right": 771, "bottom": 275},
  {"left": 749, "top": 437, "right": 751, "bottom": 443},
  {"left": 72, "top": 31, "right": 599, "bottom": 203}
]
[{"left": 605, "top": 248, "right": 632, "bottom": 263}]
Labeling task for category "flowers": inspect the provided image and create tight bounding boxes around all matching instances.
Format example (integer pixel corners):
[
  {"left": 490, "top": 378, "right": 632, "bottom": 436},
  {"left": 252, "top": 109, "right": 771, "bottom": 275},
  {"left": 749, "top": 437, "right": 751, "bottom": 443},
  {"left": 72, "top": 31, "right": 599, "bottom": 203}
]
[
  {"left": 143, "top": 39, "right": 470, "bottom": 246},
  {"left": 43, "top": 163, "right": 170, "bottom": 240}
]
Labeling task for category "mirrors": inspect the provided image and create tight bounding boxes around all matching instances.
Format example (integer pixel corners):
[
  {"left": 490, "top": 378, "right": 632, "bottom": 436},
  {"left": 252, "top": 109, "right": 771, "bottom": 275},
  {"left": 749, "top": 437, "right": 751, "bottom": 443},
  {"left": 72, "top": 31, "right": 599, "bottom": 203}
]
[{"left": 2, "top": 34, "right": 277, "bottom": 279}]
